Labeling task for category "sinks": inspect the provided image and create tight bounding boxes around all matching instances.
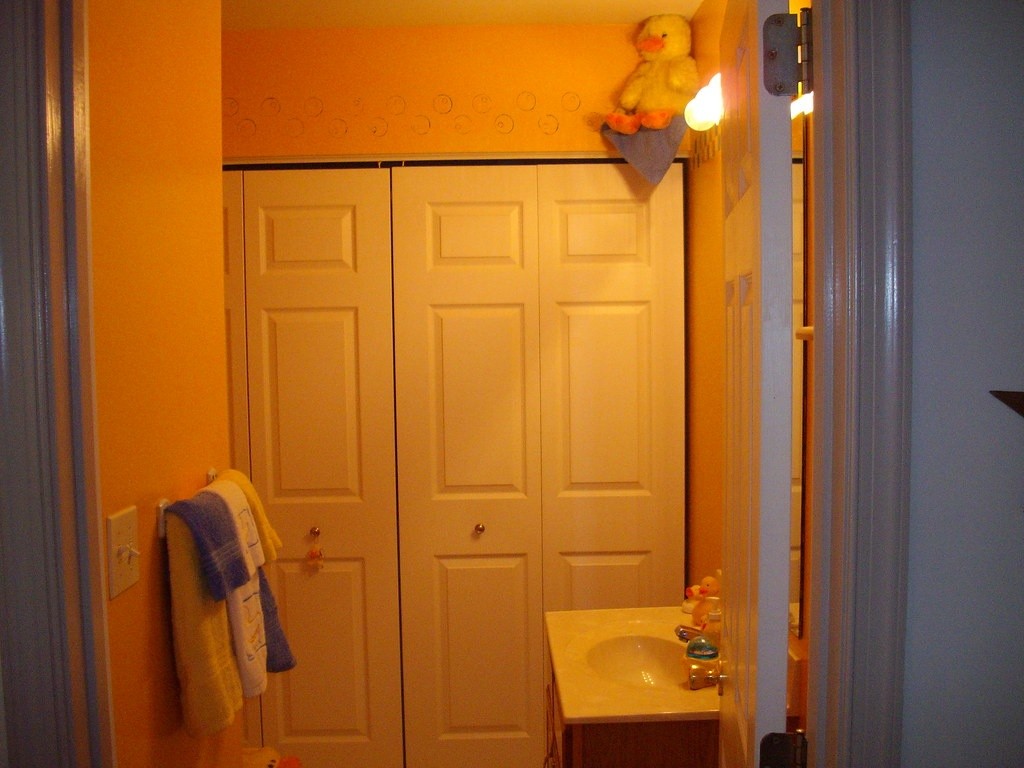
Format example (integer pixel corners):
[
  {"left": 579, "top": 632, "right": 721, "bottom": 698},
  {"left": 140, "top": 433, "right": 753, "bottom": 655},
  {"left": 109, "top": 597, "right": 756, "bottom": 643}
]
[{"left": 563, "top": 621, "right": 703, "bottom": 702}]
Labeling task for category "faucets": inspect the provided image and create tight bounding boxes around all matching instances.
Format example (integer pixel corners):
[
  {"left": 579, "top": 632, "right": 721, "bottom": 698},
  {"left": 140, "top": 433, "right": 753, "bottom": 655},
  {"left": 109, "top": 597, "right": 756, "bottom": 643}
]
[{"left": 673, "top": 625, "right": 703, "bottom": 643}]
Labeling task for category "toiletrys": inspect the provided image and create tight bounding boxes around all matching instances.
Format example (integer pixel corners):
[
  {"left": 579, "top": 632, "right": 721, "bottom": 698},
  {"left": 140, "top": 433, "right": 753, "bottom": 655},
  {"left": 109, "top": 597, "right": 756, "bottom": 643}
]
[{"left": 702, "top": 596, "right": 721, "bottom": 648}]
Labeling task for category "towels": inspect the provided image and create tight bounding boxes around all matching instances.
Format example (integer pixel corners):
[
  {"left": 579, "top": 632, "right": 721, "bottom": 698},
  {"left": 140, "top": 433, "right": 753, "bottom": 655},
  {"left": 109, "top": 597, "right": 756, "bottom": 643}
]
[{"left": 165, "top": 469, "right": 297, "bottom": 739}]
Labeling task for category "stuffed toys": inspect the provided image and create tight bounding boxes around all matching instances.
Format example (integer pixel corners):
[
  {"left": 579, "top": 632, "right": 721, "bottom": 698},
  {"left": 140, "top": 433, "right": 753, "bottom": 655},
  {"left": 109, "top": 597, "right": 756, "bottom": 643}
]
[{"left": 602, "top": 14, "right": 701, "bottom": 136}]
